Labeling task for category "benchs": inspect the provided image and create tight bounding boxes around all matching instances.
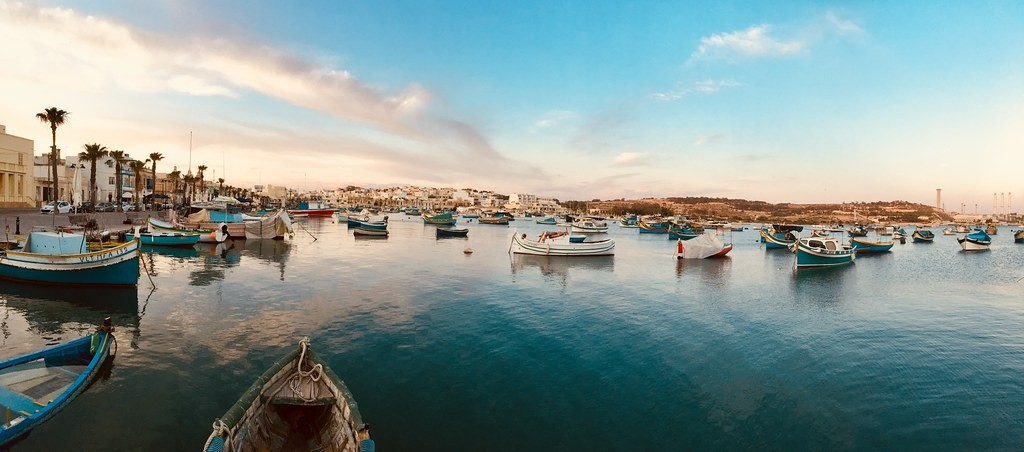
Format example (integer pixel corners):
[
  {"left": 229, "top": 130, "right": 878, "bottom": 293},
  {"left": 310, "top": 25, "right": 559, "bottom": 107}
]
[
  {"left": 158, "top": 212, "right": 169, "bottom": 222},
  {"left": 125, "top": 212, "right": 145, "bottom": 226},
  {"left": 68, "top": 215, "right": 91, "bottom": 231}
]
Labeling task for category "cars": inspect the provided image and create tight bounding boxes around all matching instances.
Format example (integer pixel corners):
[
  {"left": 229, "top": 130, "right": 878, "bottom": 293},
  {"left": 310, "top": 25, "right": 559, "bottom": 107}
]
[
  {"left": 40, "top": 200, "right": 74, "bottom": 214},
  {"left": 114, "top": 201, "right": 175, "bottom": 211},
  {"left": 95, "top": 202, "right": 115, "bottom": 212},
  {"left": 77, "top": 202, "right": 95, "bottom": 213}
]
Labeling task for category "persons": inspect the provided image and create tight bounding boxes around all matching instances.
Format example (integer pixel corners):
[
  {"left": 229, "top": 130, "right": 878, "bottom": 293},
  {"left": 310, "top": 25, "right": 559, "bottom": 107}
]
[{"left": 221, "top": 225, "right": 232, "bottom": 239}]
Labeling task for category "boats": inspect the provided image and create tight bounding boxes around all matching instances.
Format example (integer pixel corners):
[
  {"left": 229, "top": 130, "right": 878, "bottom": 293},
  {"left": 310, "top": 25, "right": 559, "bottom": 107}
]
[
  {"left": 148, "top": 216, "right": 230, "bottom": 243},
  {"left": 0, "top": 315, "right": 115, "bottom": 447},
  {"left": 126, "top": 231, "right": 201, "bottom": 247},
  {"left": 788, "top": 227, "right": 858, "bottom": 269},
  {"left": 911, "top": 230, "right": 934, "bottom": 242},
  {"left": 620, "top": 211, "right": 908, "bottom": 250},
  {"left": 676, "top": 232, "right": 732, "bottom": 260},
  {"left": 957, "top": 228, "right": 992, "bottom": 251},
  {"left": 198, "top": 334, "right": 375, "bottom": 452},
  {"left": 511, "top": 231, "right": 616, "bottom": 257},
  {"left": 0, "top": 228, "right": 144, "bottom": 287},
  {"left": 916, "top": 222, "right": 997, "bottom": 235},
  {"left": 285, "top": 195, "right": 607, "bottom": 237},
  {"left": 173, "top": 208, "right": 246, "bottom": 238},
  {"left": 851, "top": 235, "right": 894, "bottom": 255},
  {"left": 1014, "top": 230, "right": 1024, "bottom": 243}
]
